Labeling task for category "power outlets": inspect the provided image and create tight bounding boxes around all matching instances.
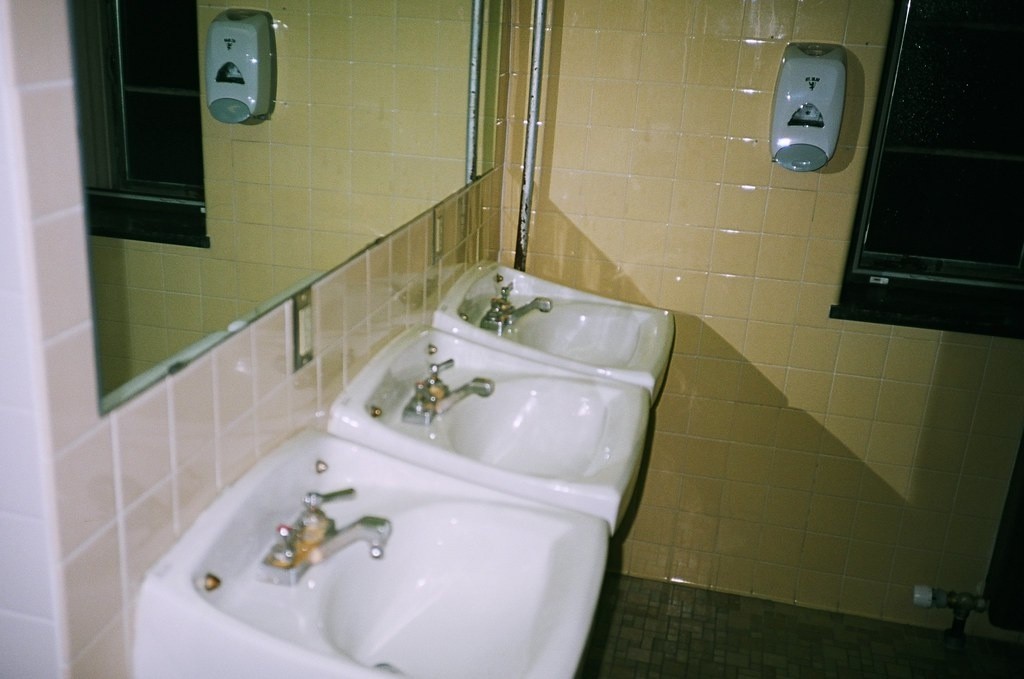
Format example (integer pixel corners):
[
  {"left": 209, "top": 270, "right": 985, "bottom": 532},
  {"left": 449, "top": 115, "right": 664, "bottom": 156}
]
[
  {"left": 434, "top": 205, "right": 446, "bottom": 257},
  {"left": 287, "top": 286, "right": 314, "bottom": 373}
]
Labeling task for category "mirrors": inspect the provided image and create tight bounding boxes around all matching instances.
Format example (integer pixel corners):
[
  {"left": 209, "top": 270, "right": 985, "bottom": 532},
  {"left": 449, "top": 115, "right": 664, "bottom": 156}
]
[{"left": 65, "top": 0, "right": 509, "bottom": 418}]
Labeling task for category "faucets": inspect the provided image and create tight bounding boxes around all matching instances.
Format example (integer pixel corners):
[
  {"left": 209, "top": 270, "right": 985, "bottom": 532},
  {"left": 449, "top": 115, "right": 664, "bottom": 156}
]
[
  {"left": 294, "top": 487, "right": 393, "bottom": 567},
  {"left": 492, "top": 297, "right": 554, "bottom": 326},
  {"left": 429, "top": 376, "right": 494, "bottom": 419}
]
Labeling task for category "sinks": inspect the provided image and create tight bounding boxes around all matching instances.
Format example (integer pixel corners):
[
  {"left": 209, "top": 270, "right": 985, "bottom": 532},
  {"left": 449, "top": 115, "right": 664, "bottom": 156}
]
[
  {"left": 126, "top": 425, "right": 613, "bottom": 679},
  {"left": 430, "top": 258, "right": 675, "bottom": 409},
  {"left": 326, "top": 320, "right": 652, "bottom": 538}
]
